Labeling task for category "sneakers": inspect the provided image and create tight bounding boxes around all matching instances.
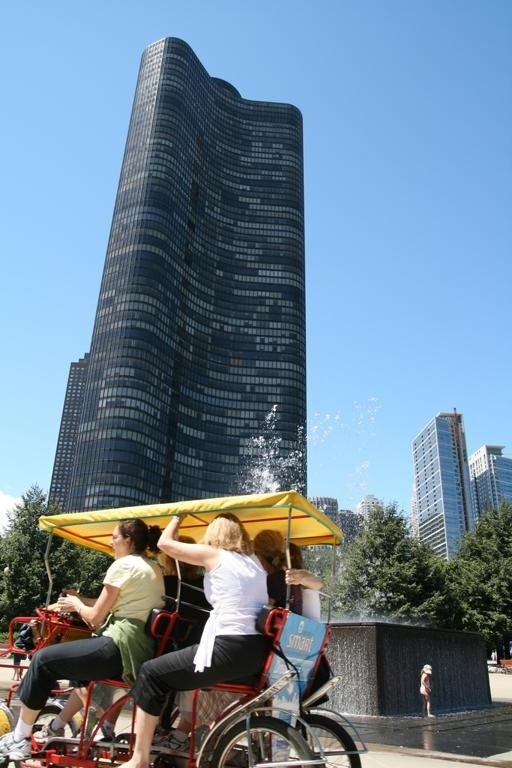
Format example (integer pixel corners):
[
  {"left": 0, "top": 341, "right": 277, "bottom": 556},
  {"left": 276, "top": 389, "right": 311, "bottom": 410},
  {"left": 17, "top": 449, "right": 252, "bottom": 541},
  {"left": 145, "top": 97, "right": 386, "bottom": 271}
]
[
  {"left": 32, "top": 718, "right": 66, "bottom": 744},
  {"left": 0, "top": 732, "right": 32, "bottom": 764},
  {"left": 151, "top": 732, "right": 190, "bottom": 754}
]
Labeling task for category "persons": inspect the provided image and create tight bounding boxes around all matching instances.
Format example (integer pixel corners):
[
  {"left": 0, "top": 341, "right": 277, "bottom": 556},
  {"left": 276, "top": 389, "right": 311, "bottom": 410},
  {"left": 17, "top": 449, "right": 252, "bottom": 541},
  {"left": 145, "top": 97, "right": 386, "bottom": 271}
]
[{"left": 419, "top": 664, "right": 436, "bottom": 717}]
